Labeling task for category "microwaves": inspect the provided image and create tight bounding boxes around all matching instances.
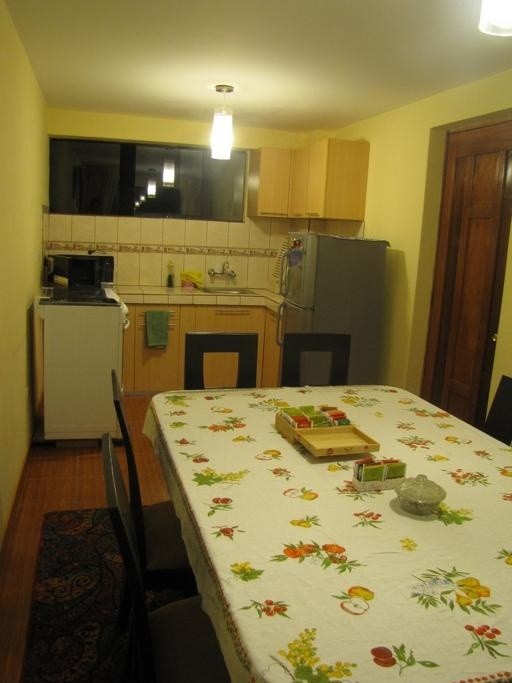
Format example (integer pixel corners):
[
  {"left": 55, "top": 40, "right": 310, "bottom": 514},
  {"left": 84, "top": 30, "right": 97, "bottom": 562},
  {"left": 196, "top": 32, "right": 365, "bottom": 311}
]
[{"left": 43, "top": 252, "right": 113, "bottom": 296}]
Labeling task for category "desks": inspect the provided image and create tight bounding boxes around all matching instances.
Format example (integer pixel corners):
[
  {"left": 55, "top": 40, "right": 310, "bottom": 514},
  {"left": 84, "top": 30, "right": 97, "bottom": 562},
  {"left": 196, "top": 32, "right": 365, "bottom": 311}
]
[{"left": 144, "top": 384, "right": 512, "bottom": 683}]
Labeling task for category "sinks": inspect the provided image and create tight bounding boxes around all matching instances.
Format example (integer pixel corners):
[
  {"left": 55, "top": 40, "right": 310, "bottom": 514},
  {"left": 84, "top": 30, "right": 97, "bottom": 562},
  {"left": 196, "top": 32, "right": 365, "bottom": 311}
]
[{"left": 201, "top": 286, "right": 258, "bottom": 296}]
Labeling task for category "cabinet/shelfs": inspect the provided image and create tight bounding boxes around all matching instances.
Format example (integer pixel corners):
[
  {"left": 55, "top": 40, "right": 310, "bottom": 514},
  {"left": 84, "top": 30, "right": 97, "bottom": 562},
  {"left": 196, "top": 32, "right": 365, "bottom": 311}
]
[
  {"left": 123, "top": 304, "right": 282, "bottom": 392},
  {"left": 247, "top": 138, "right": 370, "bottom": 221}
]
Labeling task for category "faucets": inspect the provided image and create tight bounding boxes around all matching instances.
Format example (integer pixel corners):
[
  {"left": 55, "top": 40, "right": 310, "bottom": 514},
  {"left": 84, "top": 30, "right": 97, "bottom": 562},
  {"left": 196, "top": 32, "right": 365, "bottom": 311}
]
[{"left": 221, "top": 261, "right": 230, "bottom": 274}]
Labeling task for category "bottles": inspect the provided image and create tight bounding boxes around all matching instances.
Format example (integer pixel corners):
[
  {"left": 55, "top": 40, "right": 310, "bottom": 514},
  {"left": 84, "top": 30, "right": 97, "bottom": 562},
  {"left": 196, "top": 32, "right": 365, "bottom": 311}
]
[{"left": 167, "top": 259, "right": 175, "bottom": 289}]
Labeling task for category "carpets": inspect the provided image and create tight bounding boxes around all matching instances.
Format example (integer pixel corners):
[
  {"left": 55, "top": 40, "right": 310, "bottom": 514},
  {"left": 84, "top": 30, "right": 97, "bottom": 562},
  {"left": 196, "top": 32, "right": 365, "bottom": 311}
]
[{"left": 21, "top": 506, "right": 183, "bottom": 683}]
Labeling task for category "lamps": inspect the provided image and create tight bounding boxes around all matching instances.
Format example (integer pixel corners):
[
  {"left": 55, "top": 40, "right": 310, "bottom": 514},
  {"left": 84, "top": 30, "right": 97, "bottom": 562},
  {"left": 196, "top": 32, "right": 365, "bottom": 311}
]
[
  {"left": 162, "top": 147, "right": 177, "bottom": 187},
  {"left": 147, "top": 169, "right": 156, "bottom": 198},
  {"left": 210, "top": 85, "right": 234, "bottom": 160},
  {"left": 479, "top": 0, "right": 512, "bottom": 37}
]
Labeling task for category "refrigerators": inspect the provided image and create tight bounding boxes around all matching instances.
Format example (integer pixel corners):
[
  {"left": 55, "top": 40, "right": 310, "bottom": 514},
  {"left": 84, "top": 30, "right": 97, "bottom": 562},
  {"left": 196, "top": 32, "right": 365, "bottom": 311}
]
[{"left": 275, "top": 232, "right": 387, "bottom": 386}]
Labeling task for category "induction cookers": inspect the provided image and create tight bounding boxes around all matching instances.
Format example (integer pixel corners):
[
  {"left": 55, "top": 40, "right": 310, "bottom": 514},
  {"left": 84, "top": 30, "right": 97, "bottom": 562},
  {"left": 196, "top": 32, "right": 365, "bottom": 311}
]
[{"left": 38, "top": 291, "right": 119, "bottom": 305}]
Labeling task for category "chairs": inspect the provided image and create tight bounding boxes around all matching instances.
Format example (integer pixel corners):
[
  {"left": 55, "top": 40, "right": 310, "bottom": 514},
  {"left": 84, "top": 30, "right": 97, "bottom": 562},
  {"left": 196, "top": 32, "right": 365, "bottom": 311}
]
[
  {"left": 101, "top": 433, "right": 231, "bottom": 683},
  {"left": 484, "top": 375, "right": 512, "bottom": 446},
  {"left": 283, "top": 333, "right": 352, "bottom": 387},
  {"left": 112, "top": 369, "right": 196, "bottom": 600}
]
